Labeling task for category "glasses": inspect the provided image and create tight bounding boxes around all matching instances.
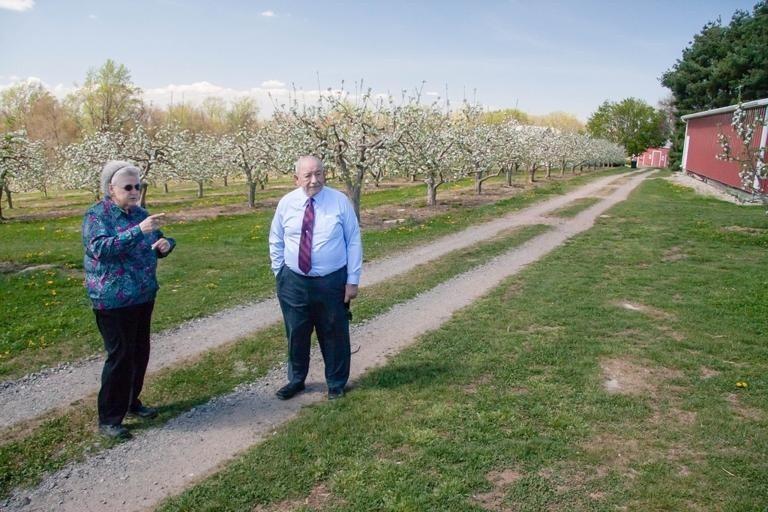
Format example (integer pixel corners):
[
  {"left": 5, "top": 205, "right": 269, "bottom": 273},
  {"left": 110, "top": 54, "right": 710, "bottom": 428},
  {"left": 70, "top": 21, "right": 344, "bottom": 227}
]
[
  {"left": 109, "top": 183, "right": 141, "bottom": 191},
  {"left": 344, "top": 300, "right": 352, "bottom": 321}
]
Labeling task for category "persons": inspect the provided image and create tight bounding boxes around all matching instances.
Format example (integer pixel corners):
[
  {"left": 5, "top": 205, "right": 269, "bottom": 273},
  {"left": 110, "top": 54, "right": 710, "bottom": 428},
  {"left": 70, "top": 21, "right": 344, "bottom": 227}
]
[
  {"left": 266, "top": 154, "right": 366, "bottom": 400},
  {"left": 81, "top": 160, "right": 177, "bottom": 441}
]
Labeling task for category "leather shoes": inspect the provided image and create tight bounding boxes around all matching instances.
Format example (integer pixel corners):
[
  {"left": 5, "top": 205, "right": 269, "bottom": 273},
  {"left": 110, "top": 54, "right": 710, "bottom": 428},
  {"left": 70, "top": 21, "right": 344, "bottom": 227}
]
[
  {"left": 97, "top": 423, "right": 129, "bottom": 441},
  {"left": 327, "top": 386, "right": 346, "bottom": 400},
  {"left": 126, "top": 407, "right": 157, "bottom": 418},
  {"left": 276, "top": 381, "right": 305, "bottom": 401}
]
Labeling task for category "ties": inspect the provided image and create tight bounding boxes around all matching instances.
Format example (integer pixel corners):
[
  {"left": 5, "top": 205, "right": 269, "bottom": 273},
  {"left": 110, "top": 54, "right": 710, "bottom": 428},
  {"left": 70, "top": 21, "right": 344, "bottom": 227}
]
[{"left": 297, "top": 197, "right": 315, "bottom": 275}]
